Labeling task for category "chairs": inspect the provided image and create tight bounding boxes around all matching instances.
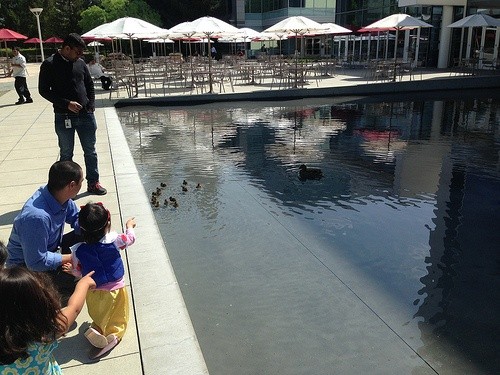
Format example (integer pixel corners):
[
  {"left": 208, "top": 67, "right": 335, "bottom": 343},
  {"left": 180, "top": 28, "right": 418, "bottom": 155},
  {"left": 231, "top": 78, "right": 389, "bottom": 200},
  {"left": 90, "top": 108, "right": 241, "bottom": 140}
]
[
  {"left": 447, "top": 56, "right": 497, "bottom": 77},
  {"left": 93, "top": 52, "right": 349, "bottom": 98},
  {"left": 361, "top": 56, "right": 422, "bottom": 83}
]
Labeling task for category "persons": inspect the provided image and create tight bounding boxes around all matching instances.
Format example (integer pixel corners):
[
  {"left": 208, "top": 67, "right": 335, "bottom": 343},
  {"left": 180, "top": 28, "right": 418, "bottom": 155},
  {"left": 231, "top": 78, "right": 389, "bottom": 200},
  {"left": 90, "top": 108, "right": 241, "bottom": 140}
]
[
  {"left": 38, "top": 33, "right": 107, "bottom": 194},
  {"left": 11, "top": 47, "right": 33, "bottom": 104},
  {"left": 89, "top": 58, "right": 112, "bottom": 91},
  {"left": 6, "top": 160, "right": 83, "bottom": 299},
  {"left": 0, "top": 241, "right": 96, "bottom": 375},
  {"left": 237, "top": 49, "right": 243, "bottom": 57},
  {"left": 212, "top": 46, "right": 217, "bottom": 58},
  {"left": 62, "top": 201, "right": 136, "bottom": 358}
]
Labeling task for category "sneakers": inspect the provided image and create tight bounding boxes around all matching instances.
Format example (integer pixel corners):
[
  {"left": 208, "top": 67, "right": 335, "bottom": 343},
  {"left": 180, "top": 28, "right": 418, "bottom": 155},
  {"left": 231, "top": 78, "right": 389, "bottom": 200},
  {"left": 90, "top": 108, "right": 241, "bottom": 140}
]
[{"left": 87, "top": 183, "right": 107, "bottom": 195}]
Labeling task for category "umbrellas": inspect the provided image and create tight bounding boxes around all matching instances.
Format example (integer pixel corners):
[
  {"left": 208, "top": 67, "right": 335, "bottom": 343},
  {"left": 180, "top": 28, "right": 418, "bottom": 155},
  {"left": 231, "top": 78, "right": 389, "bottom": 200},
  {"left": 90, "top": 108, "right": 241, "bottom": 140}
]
[
  {"left": 44, "top": 37, "right": 64, "bottom": 47},
  {"left": 447, "top": 12, "right": 500, "bottom": 75},
  {"left": 24, "top": 37, "right": 45, "bottom": 50},
  {"left": 358, "top": 14, "right": 434, "bottom": 81},
  {"left": 0, "top": 28, "right": 28, "bottom": 71},
  {"left": 81, "top": 15, "right": 352, "bottom": 92}
]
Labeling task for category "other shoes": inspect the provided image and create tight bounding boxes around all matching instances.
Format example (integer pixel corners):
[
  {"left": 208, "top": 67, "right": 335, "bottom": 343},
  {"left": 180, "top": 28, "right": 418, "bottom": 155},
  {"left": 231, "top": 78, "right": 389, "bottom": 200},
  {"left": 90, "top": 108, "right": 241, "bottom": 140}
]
[
  {"left": 84, "top": 327, "right": 108, "bottom": 348},
  {"left": 89, "top": 335, "right": 117, "bottom": 360},
  {"left": 25, "top": 97, "right": 33, "bottom": 102},
  {"left": 15, "top": 100, "right": 25, "bottom": 104}
]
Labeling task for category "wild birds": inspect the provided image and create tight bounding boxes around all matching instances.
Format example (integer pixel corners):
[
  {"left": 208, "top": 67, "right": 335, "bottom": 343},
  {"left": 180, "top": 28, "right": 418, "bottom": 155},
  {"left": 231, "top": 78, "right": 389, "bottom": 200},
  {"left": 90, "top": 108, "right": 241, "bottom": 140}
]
[
  {"left": 151, "top": 179, "right": 201, "bottom": 207},
  {"left": 299, "top": 163, "right": 322, "bottom": 178}
]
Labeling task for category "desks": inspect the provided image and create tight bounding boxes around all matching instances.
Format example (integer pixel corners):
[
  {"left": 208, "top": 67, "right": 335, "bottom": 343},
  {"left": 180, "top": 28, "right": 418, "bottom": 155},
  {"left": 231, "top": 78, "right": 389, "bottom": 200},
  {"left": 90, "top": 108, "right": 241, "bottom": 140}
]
[
  {"left": 243, "top": 64, "right": 265, "bottom": 84},
  {"left": 192, "top": 72, "right": 212, "bottom": 93},
  {"left": 289, "top": 68, "right": 304, "bottom": 88},
  {"left": 124, "top": 74, "right": 147, "bottom": 98}
]
